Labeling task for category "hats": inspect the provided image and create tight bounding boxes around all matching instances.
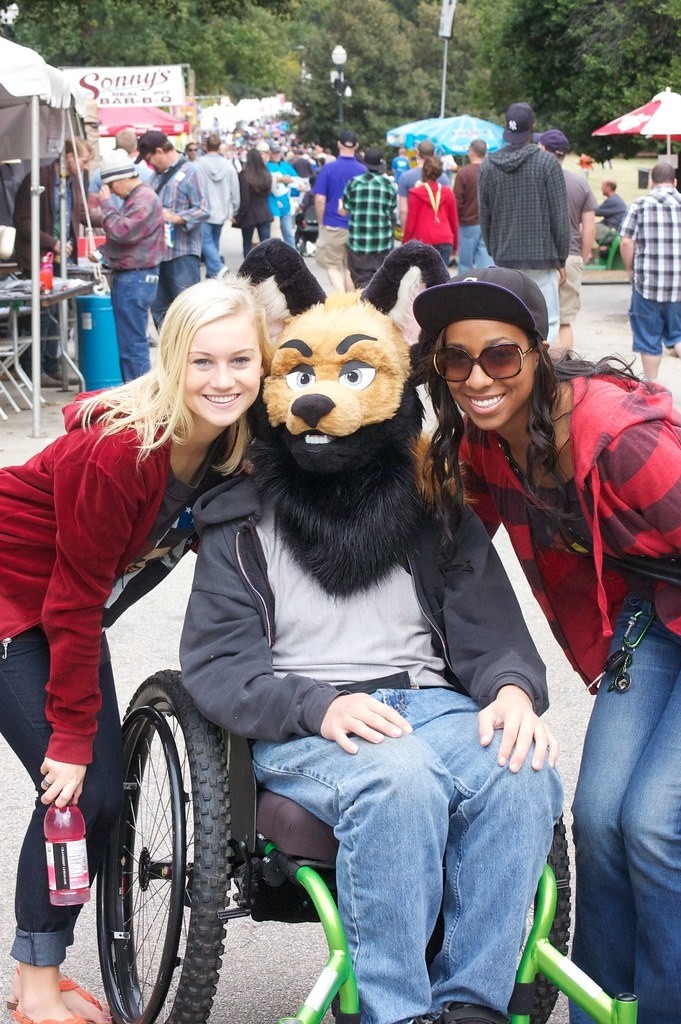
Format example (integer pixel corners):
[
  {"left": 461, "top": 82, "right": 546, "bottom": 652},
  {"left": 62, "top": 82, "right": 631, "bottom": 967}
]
[
  {"left": 533, "top": 129, "right": 570, "bottom": 156},
  {"left": 255, "top": 142, "right": 269, "bottom": 152},
  {"left": 339, "top": 130, "right": 359, "bottom": 148},
  {"left": 503, "top": 102, "right": 535, "bottom": 145},
  {"left": 270, "top": 141, "right": 282, "bottom": 152},
  {"left": 98, "top": 149, "right": 138, "bottom": 184},
  {"left": 363, "top": 149, "right": 384, "bottom": 170},
  {"left": 133, "top": 130, "right": 166, "bottom": 165},
  {"left": 413, "top": 266, "right": 550, "bottom": 341}
]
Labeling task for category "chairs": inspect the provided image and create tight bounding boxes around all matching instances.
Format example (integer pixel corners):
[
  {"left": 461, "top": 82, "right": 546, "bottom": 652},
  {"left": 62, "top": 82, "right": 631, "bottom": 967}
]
[{"left": 0, "top": 339, "right": 47, "bottom": 421}]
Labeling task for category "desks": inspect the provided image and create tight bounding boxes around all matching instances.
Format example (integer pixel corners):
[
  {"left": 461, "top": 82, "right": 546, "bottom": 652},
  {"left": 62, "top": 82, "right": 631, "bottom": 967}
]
[{"left": 0, "top": 280, "right": 95, "bottom": 421}]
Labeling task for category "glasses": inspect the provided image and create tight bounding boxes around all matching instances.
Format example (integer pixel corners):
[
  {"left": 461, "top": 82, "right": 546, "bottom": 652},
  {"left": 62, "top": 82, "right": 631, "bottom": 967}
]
[
  {"left": 145, "top": 152, "right": 154, "bottom": 166},
  {"left": 188, "top": 149, "right": 199, "bottom": 152},
  {"left": 432, "top": 338, "right": 538, "bottom": 382}
]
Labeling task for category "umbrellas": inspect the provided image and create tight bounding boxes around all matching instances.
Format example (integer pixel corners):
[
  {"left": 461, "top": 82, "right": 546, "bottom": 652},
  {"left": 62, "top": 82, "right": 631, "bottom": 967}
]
[
  {"left": 406, "top": 114, "right": 511, "bottom": 166},
  {"left": 591, "top": 87, "right": 681, "bottom": 163},
  {"left": 386, "top": 120, "right": 427, "bottom": 148}
]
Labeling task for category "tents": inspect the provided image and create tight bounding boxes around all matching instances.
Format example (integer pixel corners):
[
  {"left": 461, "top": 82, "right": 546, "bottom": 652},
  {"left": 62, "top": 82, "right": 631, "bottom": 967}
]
[
  {"left": 0, "top": 35, "right": 104, "bottom": 440},
  {"left": 97, "top": 106, "right": 190, "bottom": 137}
]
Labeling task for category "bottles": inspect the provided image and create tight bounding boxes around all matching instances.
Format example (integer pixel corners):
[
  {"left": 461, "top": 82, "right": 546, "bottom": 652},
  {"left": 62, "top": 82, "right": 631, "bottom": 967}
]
[
  {"left": 40, "top": 252, "right": 53, "bottom": 293},
  {"left": 43, "top": 802, "right": 90, "bottom": 906}
]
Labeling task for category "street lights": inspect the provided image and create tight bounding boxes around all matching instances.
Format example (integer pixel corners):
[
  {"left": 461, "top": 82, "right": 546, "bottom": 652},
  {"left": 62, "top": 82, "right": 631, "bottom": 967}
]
[{"left": 327, "top": 42, "right": 354, "bottom": 135}]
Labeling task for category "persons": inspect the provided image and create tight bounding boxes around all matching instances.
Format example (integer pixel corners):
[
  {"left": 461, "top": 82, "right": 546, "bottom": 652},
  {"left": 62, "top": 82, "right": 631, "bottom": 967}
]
[
  {"left": 477, "top": 101, "right": 572, "bottom": 352},
  {"left": 179, "top": 238, "right": 564, "bottom": 1024},
  {"left": 91, "top": 127, "right": 158, "bottom": 349},
  {"left": 413, "top": 265, "right": 681, "bottom": 1024},
  {"left": 619, "top": 162, "right": 681, "bottom": 381},
  {"left": 102, "top": 156, "right": 165, "bottom": 385},
  {"left": 534, "top": 130, "right": 600, "bottom": 352},
  {"left": 186, "top": 118, "right": 494, "bottom": 295},
  {"left": 12, "top": 137, "right": 102, "bottom": 387},
  {"left": 0, "top": 279, "right": 272, "bottom": 1024},
  {"left": 580, "top": 180, "right": 626, "bottom": 265},
  {"left": 134, "top": 130, "right": 211, "bottom": 336}
]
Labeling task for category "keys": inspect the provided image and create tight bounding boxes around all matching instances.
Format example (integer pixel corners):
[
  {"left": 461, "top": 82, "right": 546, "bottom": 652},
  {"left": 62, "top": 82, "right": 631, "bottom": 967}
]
[{"left": 585, "top": 649, "right": 632, "bottom": 694}]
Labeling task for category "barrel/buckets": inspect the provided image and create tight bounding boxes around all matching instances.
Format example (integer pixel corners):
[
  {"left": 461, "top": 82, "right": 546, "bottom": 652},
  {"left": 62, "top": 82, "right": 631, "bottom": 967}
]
[
  {"left": 75, "top": 291, "right": 124, "bottom": 392},
  {"left": 78, "top": 236, "right": 108, "bottom": 256}
]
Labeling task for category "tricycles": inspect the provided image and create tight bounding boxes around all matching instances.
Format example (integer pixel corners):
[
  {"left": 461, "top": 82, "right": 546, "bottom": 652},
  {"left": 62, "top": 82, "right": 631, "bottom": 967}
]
[{"left": 95, "top": 670, "right": 650, "bottom": 1024}]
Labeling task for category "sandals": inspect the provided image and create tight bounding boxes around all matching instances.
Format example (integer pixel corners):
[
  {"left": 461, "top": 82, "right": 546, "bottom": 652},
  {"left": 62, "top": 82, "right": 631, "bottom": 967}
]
[{"left": 6, "top": 966, "right": 112, "bottom": 1024}]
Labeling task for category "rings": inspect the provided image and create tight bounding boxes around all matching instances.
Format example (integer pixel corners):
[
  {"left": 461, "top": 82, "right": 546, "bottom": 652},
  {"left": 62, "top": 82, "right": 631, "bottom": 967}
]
[{"left": 43, "top": 779, "right": 52, "bottom": 786}]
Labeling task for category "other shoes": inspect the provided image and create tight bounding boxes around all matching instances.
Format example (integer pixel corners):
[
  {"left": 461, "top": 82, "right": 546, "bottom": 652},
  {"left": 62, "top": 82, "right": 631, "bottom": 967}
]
[
  {"left": 30, "top": 371, "right": 63, "bottom": 388},
  {"left": 51, "top": 369, "right": 81, "bottom": 385},
  {"left": 216, "top": 267, "right": 230, "bottom": 281},
  {"left": 431, "top": 1001, "right": 512, "bottom": 1024}
]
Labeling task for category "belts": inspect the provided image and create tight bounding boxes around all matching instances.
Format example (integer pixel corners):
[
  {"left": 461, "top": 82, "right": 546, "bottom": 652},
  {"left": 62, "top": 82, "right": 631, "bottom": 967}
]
[{"left": 112, "top": 265, "right": 156, "bottom": 273}]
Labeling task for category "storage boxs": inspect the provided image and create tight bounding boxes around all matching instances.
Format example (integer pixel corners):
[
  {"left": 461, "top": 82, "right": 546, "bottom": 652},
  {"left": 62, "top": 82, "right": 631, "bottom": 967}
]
[{"left": 77, "top": 236, "right": 110, "bottom": 270}]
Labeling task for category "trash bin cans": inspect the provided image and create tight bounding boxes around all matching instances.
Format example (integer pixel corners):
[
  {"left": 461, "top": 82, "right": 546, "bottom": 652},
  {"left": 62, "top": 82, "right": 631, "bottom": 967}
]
[
  {"left": 75, "top": 295, "right": 123, "bottom": 391},
  {"left": 639, "top": 168, "right": 649, "bottom": 189}
]
